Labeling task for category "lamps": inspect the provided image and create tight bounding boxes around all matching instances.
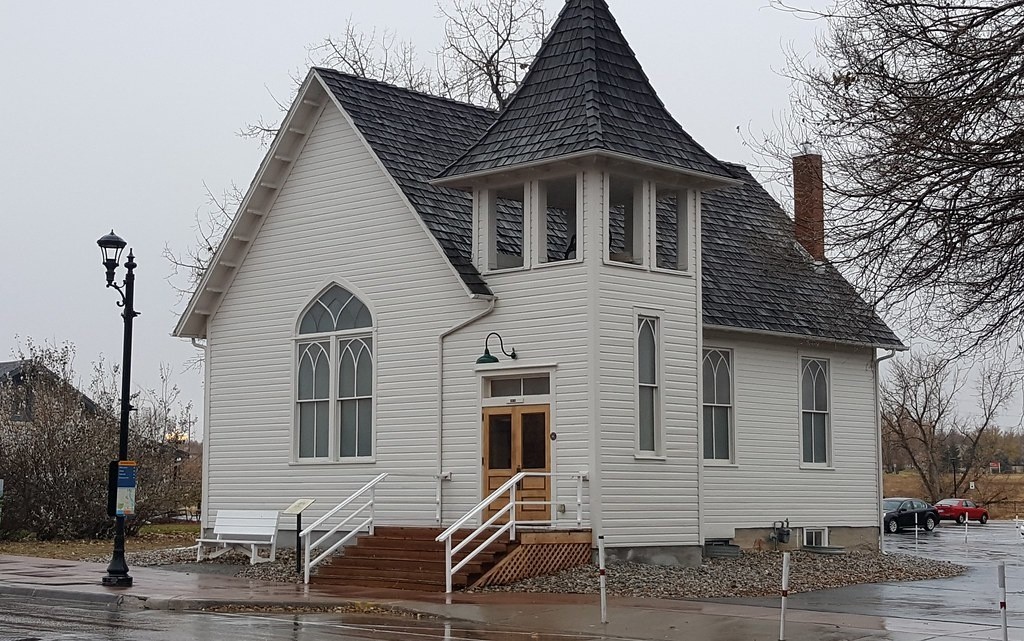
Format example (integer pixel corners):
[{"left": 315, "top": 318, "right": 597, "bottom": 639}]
[{"left": 475, "top": 331, "right": 517, "bottom": 364}]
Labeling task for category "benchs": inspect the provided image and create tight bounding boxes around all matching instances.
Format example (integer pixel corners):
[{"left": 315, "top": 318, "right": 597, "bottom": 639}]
[{"left": 194, "top": 509, "right": 281, "bottom": 566}]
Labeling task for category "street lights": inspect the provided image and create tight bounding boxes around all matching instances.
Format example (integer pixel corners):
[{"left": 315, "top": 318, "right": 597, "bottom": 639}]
[
  {"left": 950, "top": 454, "right": 957, "bottom": 497},
  {"left": 96, "top": 229, "right": 134, "bottom": 590}
]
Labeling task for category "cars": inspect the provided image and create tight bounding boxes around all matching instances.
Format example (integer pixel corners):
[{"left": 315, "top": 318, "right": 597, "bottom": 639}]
[
  {"left": 934, "top": 498, "right": 990, "bottom": 524},
  {"left": 882, "top": 497, "right": 941, "bottom": 534}
]
